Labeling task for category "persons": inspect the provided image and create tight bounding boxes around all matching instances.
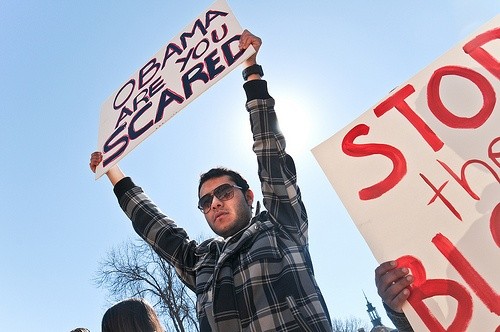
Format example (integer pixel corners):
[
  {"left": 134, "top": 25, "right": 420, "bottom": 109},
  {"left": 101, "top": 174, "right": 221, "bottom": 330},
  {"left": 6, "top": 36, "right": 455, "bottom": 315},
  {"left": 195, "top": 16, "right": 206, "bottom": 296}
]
[
  {"left": 90, "top": 28, "right": 333, "bottom": 332},
  {"left": 375, "top": 259, "right": 414, "bottom": 332}
]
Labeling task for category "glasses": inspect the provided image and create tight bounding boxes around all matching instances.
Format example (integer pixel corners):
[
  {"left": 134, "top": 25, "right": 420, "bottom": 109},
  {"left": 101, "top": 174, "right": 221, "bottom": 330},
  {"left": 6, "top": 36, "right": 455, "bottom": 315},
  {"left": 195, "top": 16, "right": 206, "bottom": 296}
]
[{"left": 198, "top": 183, "right": 246, "bottom": 214}]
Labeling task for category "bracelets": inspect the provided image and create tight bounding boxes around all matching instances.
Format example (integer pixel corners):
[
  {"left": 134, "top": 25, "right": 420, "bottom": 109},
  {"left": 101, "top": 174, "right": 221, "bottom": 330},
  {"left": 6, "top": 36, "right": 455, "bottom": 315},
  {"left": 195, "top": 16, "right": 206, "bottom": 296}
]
[{"left": 241, "top": 63, "right": 267, "bottom": 81}]
